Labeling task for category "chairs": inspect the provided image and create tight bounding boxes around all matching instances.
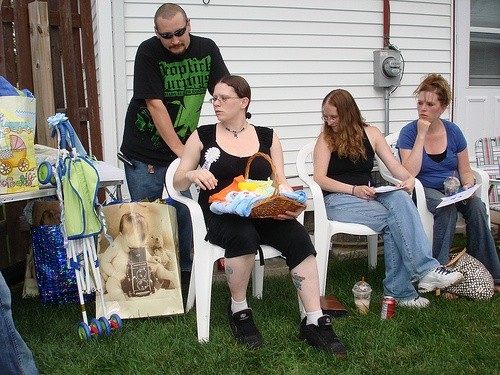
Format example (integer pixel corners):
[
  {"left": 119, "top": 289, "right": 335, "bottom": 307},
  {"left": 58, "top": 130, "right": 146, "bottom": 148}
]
[
  {"left": 474, "top": 136, "right": 500, "bottom": 225},
  {"left": 296, "top": 140, "right": 413, "bottom": 299},
  {"left": 165, "top": 156, "right": 306, "bottom": 345},
  {"left": 374, "top": 133, "right": 491, "bottom": 258}
]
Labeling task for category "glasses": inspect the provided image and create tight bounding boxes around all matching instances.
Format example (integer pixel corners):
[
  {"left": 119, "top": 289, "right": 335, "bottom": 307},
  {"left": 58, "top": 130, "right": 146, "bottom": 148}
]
[
  {"left": 157, "top": 21, "right": 188, "bottom": 39},
  {"left": 210, "top": 96, "right": 242, "bottom": 103},
  {"left": 321, "top": 115, "right": 339, "bottom": 122}
]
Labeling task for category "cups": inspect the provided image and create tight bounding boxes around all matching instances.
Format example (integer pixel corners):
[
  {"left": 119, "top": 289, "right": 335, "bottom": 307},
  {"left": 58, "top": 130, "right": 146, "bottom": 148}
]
[
  {"left": 352, "top": 281, "right": 373, "bottom": 315},
  {"left": 443, "top": 176, "right": 461, "bottom": 197}
]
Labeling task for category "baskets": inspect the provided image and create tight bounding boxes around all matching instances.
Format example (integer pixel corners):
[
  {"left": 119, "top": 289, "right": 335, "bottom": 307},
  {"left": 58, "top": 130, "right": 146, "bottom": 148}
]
[{"left": 246, "top": 152, "right": 305, "bottom": 219}]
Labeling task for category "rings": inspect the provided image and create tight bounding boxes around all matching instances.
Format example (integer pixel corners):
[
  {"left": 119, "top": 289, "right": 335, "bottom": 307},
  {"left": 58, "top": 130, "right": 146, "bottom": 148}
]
[{"left": 365, "top": 190, "right": 366, "bottom": 194}]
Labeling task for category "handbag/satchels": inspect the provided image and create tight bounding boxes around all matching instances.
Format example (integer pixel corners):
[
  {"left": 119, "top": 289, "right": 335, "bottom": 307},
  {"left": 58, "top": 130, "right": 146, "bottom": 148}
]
[
  {"left": 94, "top": 202, "right": 185, "bottom": 322},
  {"left": 441, "top": 246, "right": 494, "bottom": 300},
  {"left": 0, "top": 87, "right": 39, "bottom": 194},
  {"left": 22, "top": 199, "right": 93, "bottom": 307}
]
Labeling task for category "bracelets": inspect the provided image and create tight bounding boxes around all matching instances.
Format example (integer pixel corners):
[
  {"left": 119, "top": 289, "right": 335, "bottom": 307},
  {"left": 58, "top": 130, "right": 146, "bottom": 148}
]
[
  {"left": 464, "top": 183, "right": 473, "bottom": 186},
  {"left": 352, "top": 185, "right": 356, "bottom": 195}
]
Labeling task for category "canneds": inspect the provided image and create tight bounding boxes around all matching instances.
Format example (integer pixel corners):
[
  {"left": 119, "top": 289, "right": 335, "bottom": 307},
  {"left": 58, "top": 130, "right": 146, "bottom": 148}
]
[{"left": 381, "top": 296, "right": 395, "bottom": 321}]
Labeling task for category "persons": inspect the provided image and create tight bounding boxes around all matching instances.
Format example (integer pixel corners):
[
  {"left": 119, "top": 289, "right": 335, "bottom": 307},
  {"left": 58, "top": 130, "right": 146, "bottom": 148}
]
[
  {"left": 172, "top": 76, "right": 346, "bottom": 353},
  {"left": 314, "top": 90, "right": 464, "bottom": 307},
  {"left": 98, "top": 213, "right": 174, "bottom": 299},
  {"left": 396, "top": 73, "right": 500, "bottom": 299},
  {"left": 0, "top": 272, "right": 39, "bottom": 375},
  {"left": 120, "top": 4, "right": 233, "bottom": 284}
]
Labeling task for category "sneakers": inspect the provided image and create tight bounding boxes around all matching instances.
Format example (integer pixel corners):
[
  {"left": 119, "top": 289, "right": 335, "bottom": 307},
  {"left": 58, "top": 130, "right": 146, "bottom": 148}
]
[
  {"left": 418, "top": 267, "right": 463, "bottom": 293},
  {"left": 298, "top": 313, "right": 347, "bottom": 358},
  {"left": 395, "top": 295, "right": 430, "bottom": 308},
  {"left": 227, "top": 300, "right": 261, "bottom": 347}
]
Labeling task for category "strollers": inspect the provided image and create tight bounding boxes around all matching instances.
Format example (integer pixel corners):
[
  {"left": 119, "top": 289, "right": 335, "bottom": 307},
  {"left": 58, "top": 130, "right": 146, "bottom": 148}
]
[{"left": 37, "top": 112, "right": 123, "bottom": 339}]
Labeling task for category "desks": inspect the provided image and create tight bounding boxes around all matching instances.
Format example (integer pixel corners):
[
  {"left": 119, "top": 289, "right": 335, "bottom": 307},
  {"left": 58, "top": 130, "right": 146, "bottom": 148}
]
[{"left": 0, "top": 143, "right": 125, "bottom": 207}]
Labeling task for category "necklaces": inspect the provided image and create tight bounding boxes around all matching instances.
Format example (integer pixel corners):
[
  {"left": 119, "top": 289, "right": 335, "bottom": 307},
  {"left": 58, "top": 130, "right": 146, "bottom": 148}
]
[{"left": 223, "top": 122, "right": 247, "bottom": 138}]
